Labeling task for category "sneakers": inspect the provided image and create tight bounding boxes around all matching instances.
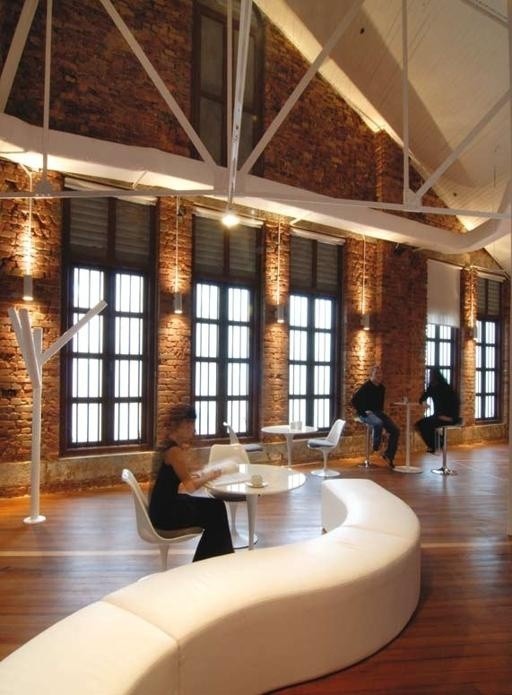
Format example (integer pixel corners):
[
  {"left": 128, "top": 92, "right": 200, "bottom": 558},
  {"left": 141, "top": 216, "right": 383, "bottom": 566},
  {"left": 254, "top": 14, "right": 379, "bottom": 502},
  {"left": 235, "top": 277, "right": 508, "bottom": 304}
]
[
  {"left": 382, "top": 455, "right": 395, "bottom": 468},
  {"left": 372, "top": 443, "right": 379, "bottom": 451}
]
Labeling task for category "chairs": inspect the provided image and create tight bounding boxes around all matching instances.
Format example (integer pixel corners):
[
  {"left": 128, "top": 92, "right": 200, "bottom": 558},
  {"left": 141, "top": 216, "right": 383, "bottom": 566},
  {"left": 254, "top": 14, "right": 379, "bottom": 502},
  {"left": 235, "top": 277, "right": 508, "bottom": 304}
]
[
  {"left": 432, "top": 417, "right": 464, "bottom": 474},
  {"left": 121, "top": 468, "right": 203, "bottom": 572},
  {"left": 354, "top": 415, "right": 377, "bottom": 468},
  {"left": 208, "top": 444, "right": 258, "bottom": 549},
  {"left": 224, "top": 422, "right": 264, "bottom": 453},
  {"left": 307, "top": 419, "right": 346, "bottom": 477}
]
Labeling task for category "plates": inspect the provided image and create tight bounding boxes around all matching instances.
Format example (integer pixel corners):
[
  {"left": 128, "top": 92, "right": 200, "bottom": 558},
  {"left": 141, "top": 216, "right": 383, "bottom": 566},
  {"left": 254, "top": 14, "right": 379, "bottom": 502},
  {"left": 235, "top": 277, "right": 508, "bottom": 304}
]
[{"left": 245, "top": 482, "right": 269, "bottom": 487}]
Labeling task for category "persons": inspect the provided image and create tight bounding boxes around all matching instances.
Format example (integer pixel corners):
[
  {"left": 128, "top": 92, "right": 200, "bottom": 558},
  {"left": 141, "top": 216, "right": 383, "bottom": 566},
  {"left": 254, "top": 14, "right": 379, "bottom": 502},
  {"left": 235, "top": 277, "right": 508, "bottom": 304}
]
[
  {"left": 350, "top": 367, "right": 400, "bottom": 472},
  {"left": 415, "top": 366, "right": 461, "bottom": 454},
  {"left": 145, "top": 402, "right": 234, "bottom": 563}
]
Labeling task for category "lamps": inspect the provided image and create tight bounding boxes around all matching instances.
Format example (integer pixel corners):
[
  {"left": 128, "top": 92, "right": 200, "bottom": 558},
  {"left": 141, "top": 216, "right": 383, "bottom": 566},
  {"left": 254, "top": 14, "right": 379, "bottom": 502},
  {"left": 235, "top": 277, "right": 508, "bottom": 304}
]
[
  {"left": 361, "top": 235, "right": 371, "bottom": 330},
  {"left": 274, "top": 219, "right": 285, "bottom": 323},
  {"left": 222, "top": 207, "right": 240, "bottom": 230},
  {"left": 467, "top": 273, "right": 477, "bottom": 339},
  {"left": 172, "top": 195, "right": 183, "bottom": 314},
  {"left": 20, "top": 164, "right": 33, "bottom": 304}
]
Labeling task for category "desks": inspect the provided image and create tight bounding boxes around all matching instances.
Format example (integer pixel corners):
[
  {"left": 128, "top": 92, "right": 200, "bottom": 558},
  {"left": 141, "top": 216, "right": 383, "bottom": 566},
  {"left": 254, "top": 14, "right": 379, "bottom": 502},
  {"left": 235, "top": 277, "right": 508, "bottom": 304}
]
[
  {"left": 260, "top": 425, "right": 317, "bottom": 469},
  {"left": 391, "top": 401, "right": 431, "bottom": 474}
]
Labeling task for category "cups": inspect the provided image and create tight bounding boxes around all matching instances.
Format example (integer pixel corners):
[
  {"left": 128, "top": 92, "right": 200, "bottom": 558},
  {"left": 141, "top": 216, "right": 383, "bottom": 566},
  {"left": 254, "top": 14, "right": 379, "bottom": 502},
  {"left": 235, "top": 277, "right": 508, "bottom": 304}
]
[{"left": 250, "top": 473, "right": 263, "bottom": 485}]
